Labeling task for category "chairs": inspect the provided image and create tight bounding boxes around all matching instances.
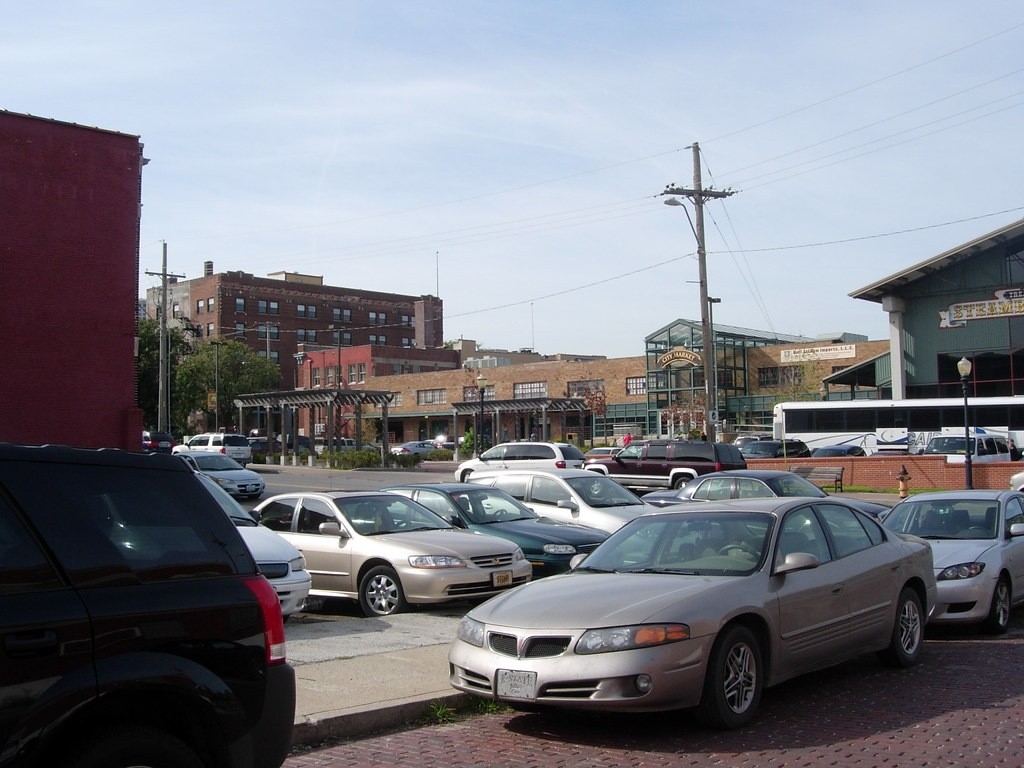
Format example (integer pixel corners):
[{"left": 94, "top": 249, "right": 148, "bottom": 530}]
[
  {"left": 368, "top": 503, "right": 396, "bottom": 530},
  {"left": 456, "top": 497, "right": 479, "bottom": 523},
  {"left": 950, "top": 510, "right": 969, "bottom": 529},
  {"left": 781, "top": 531, "right": 808, "bottom": 551},
  {"left": 691, "top": 525, "right": 728, "bottom": 559},
  {"left": 573, "top": 487, "right": 587, "bottom": 505},
  {"left": 354, "top": 503, "right": 371, "bottom": 530},
  {"left": 920, "top": 510, "right": 951, "bottom": 530},
  {"left": 743, "top": 533, "right": 786, "bottom": 561},
  {"left": 758, "top": 484, "right": 773, "bottom": 497},
  {"left": 984, "top": 507, "right": 996, "bottom": 529}
]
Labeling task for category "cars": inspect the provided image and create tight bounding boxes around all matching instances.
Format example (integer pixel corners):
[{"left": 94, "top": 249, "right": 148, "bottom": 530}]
[
  {"left": 810, "top": 445, "right": 867, "bottom": 458},
  {"left": 583, "top": 447, "right": 625, "bottom": 461},
  {"left": 641, "top": 470, "right": 894, "bottom": 536},
  {"left": 380, "top": 482, "right": 614, "bottom": 580},
  {"left": 869, "top": 450, "right": 915, "bottom": 456},
  {"left": 174, "top": 451, "right": 265, "bottom": 500},
  {"left": 246, "top": 431, "right": 311, "bottom": 452},
  {"left": 466, "top": 468, "right": 666, "bottom": 554},
  {"left": 192, "top": 468, "right": 312, "bottom": 626},
  {"left": 879, "top": 489, "right": 1024, "bottom": 636},
  {"left": 251, "top": 489, "right": 533, "bottom": 619},
  {"left": 447, "top": 496, "right": 940, "bottom": 730},
  {"left": 423, "top": 439, "right": 455, "bottom": 453},
  {"left": 391, "top": 440, "right": 440, "bottom": 461}
]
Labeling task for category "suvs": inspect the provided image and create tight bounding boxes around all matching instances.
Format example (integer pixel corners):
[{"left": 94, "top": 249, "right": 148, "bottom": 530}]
[
  {"left": 171, "top": 432, "right": 253, "bottom": 468},
  {"left": 1, "top": 440, "right": 297, "bottom": 768},
  {"left": 733, "top": 435, "right": 774, "bottom": 454},
  {"left": 581, "top": 438, "right": 747, "bottom": 490},
  {"left": 453, "top": 441, "right": 587, "bottom": 483},
  {"left": 142, "top": 430, "right": 177, "bottom": 454},
  {"left": 740, "top": 438, "right": 812, "bottom": 459}
]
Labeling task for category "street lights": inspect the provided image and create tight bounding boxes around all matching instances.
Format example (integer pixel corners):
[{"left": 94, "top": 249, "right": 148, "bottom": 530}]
[
  {"left": 957, "top": 357, "right": 974, "bottom": 490},
  {"left": 206, "top": 340, "right": 228, "bottom": 434},
  {"left": 327, "top": 324, "right": 348, "bottom": 389},
  {"left": 476, "top": 372, "right": 489, "bottom": 456},
  {"left": 707, "top": 296, "right": 722, "bottom": 431}
]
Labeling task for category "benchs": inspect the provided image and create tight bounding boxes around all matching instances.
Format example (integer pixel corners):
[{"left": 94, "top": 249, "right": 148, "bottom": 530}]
[{"left": 788, "top": 464, "right": 845, "bottom": 494}]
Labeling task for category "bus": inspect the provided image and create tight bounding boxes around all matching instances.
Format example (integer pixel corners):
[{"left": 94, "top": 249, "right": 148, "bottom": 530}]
[{"left": 771, "top": 394, "right": 1024, "bottom": 460}]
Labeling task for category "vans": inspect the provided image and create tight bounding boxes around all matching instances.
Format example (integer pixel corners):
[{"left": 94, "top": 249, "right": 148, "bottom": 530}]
[
  {"left": 315, "top": 437, "right": 357, "bottom": 456},
  {"left": 917, "top": 434, "right": 1012, "bottom": 464}
]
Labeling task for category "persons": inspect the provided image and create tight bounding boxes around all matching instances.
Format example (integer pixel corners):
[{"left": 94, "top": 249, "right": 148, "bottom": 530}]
[
  {"left": 282, "top": 506, "right": 326, "bottom": 534},
  {"left": 1008, "top": 439, "right": 1017, "bottom": 449},
  {"left": 701, "top": 432, "right": 707, "bottom": 442},
  {"left": 624, "top": 432, "right": 632, "bottom": 446},
  {"left": 530, "top": 433, "right": 539, "bottom": 441},
  {"left": 402, "top": 447, "right": 410, "bottom": 452},
  {"left": 500, "top": 423, "right": 510, "bottom": 443},
  {"left": 457, "top": 496, "right": 473, "bottom": 522}
]
